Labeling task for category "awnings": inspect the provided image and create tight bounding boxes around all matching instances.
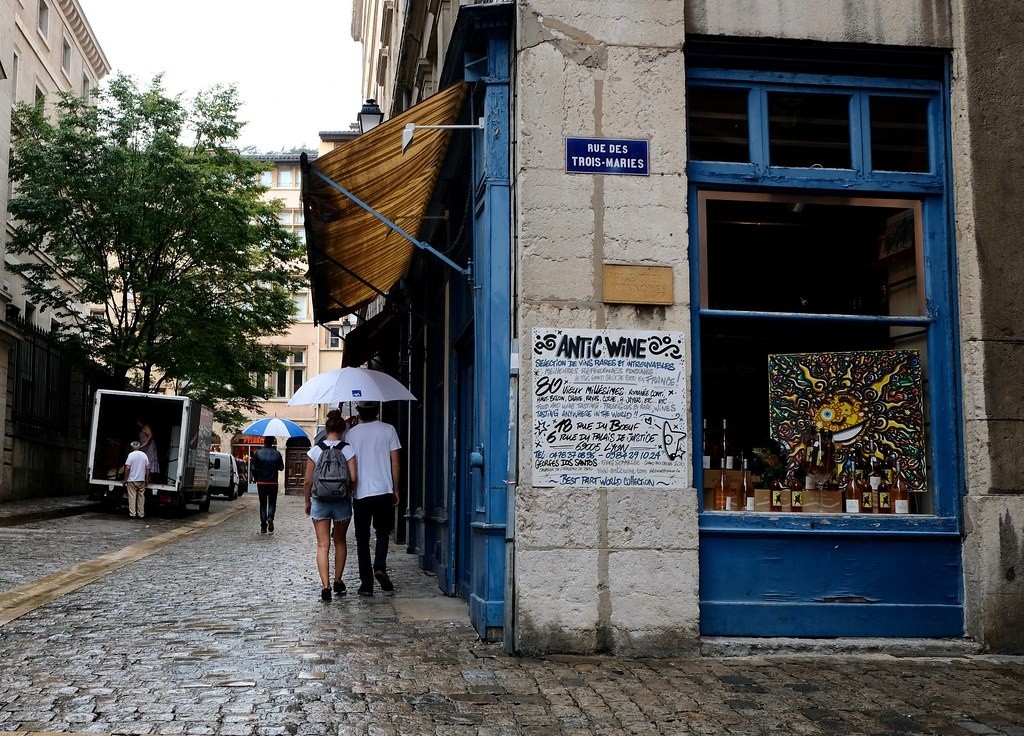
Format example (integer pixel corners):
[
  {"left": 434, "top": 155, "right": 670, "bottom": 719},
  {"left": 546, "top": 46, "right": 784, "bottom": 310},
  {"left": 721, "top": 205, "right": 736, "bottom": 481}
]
[{"left": 299, "top": 77, "right": 470, "bottom": 328}]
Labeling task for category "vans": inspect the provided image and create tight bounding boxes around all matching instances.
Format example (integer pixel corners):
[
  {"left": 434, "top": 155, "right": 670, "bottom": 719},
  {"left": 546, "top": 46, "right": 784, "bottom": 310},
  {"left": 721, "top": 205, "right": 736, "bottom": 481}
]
[{"left": 208, "top": 451, "right": 248, "bottom": 500}]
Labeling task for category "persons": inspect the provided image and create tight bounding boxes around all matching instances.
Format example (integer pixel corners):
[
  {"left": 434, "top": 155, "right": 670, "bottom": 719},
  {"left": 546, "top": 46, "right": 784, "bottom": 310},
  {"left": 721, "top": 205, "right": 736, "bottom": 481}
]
[
  {"left": 235, "top": 451, "right": 251, "bottom": 461},
  {"left": 210, "top": 446, "right": 217, "bottom": 452},
  {"left": 345, "top": 402, "right": 402, "bottom": 597},
  {"left": 137, "top": 416, "right": 160, "bottom": 483},
  {"left": 304, "top": 410, "right": 358, "bottom": 601},
  {"left": 250, "top": 436, "right": 285, "bottom": 533},
  {"left": 121, "top": 441, "right": 151, "bottom": 519}
]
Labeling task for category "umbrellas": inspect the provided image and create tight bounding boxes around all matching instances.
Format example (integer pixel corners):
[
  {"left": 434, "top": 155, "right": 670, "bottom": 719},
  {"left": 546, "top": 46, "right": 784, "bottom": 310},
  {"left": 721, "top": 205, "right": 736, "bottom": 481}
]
[
  {"left": 241, "top": 411, "right": 311, "bottom": 449},
  {"left": 284, "top": 363, "right": 418, "bottom": 446}
]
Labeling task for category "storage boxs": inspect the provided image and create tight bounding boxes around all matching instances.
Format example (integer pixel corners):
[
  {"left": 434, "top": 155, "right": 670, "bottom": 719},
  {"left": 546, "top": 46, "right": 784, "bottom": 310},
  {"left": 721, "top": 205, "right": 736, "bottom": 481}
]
[
  {"left": 704, "top": 469, "right": 751, "bottom": 511},
  {"left": 754, "top": 489, "right": 791, "bottom": 512},
  {"left": 802, "top": 490, "right": 842, "bottom": 512}
]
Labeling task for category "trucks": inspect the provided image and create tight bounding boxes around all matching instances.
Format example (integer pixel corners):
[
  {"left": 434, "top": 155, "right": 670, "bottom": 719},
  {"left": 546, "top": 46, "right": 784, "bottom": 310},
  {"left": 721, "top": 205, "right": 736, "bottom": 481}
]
[{"left": 84, "top": 388, "right": 220, "bottom": 516}]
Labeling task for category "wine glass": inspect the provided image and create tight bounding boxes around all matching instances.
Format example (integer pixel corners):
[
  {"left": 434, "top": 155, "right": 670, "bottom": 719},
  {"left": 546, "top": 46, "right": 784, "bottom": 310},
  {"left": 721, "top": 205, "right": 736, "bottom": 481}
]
[{"left": 806, "top": 439, "right": 834, "bottom": 513}]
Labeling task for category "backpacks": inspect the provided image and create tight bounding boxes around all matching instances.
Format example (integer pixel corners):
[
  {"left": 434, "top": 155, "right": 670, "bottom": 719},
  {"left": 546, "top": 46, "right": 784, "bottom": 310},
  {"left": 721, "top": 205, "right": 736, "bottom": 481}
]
[{"left": 312, "top": 441, "right": 351, "bottom": 498}]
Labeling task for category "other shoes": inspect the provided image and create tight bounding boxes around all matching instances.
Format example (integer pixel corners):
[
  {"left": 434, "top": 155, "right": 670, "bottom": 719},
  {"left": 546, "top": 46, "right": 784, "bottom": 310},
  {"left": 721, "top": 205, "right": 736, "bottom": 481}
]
[
  {"left": 357, "top": 584, "right": 373, "bottom": 596},
  {"left": 261, "top": 529, "right": 267, "bottom": 533},
  {"left": 374, "top": 569, "right": 394, "bottom": 591},
  {"left": 268, "top": 518, "right": 274, "bottom": 532},
  {"left": 334, "top": 580, "right": 346, "bottom": 592},
  {"left": 321, "top": 587, "right": 332, "bottom": 599}
]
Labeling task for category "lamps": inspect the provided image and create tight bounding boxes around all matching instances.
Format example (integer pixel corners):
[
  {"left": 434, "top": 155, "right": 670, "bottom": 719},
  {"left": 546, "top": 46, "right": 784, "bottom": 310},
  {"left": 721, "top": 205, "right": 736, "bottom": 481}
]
[
  {"left": 341, "top": 318, "right": 352, "bottom": 337},
  {"left": 357, "top": 98, "right": 385, "bottom": 135}
]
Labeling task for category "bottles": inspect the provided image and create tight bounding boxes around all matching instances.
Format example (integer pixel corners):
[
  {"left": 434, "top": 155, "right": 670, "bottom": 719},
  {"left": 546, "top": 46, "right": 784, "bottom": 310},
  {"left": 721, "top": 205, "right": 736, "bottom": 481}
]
[
  {"left": 845, "top": 461, "right": 861, "bottom": 513},
  {"left": 702, "top": 418, "right": 713, "bottom": 469},
  {"left": 878, "top": 471, "right": 890, "bottom": 514},
  {"left": 862, "top": 475, "right": 873, "bottom": 514},
  {"left": 717, "top": 419, "right": 735, "bottom": 470},
  {"left": 804, "top": 424, "right": 835, "bottom": 491},
  {"left": 890, "top": 462, "right": 910, "bottom": 514},
  {"left": 863, "top": 439, "right": 882, "bottom": 491},
  {"left": 790, "top": 469, "right": 803, "bottom": 512},
  {"left": 737, "top": 458, "right": 754, "bottom": 511},
  {"left": 770, "top": 469, "right": 782, "bottom": 512},
  {"left": 713, "top": 458, "right": 732, "bottom": 510}
]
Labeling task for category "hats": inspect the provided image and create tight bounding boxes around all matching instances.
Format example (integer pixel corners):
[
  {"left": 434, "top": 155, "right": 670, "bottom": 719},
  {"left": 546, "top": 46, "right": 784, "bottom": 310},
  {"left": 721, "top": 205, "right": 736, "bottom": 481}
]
[{"left": 357, "top": 401, "right": 378, "bottom": 408}]
